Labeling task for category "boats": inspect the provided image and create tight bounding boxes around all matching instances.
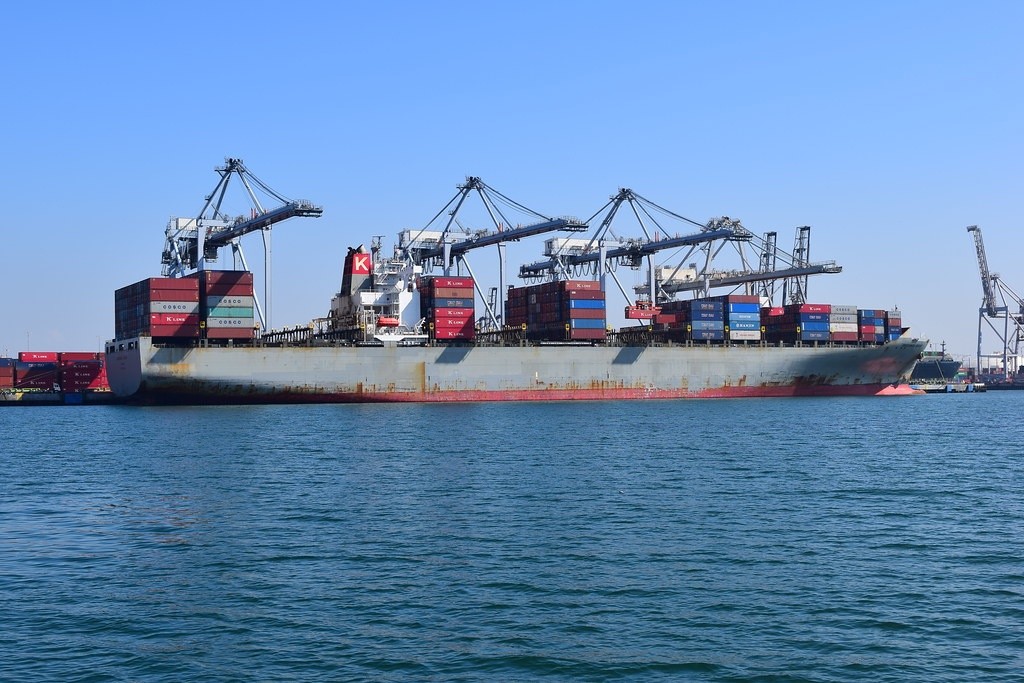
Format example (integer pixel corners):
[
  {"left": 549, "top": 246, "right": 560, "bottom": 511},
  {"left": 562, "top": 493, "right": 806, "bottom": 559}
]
[{"left": 102, "top": 157, "right": 930, "bottom": 404}]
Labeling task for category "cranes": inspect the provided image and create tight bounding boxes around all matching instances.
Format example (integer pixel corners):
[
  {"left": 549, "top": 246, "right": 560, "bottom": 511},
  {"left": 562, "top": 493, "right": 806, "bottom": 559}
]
[{"left": 965, "top": 224, "right": 1024, "bottom": 384}]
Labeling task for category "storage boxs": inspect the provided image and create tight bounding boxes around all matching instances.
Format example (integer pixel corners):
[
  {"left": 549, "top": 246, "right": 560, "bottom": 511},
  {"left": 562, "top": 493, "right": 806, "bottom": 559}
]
[{"left": 0, "top": 269, "right": 903, "bottom": 389}]
